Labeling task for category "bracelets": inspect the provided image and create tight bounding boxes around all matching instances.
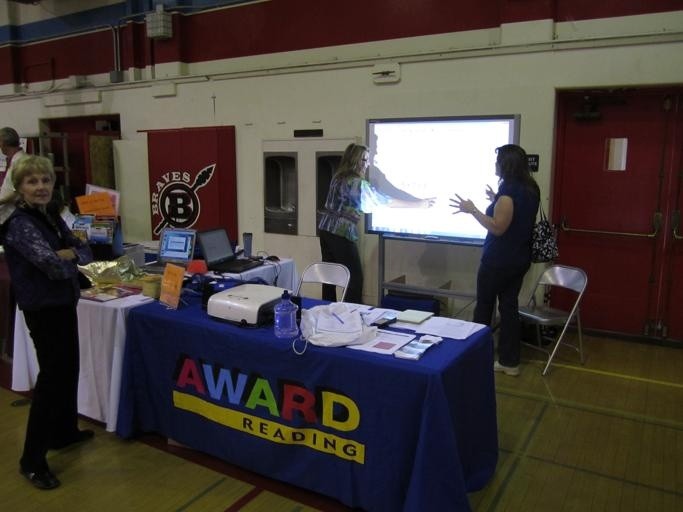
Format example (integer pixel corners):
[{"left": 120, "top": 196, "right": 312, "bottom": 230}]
[{"left": 468, "top": 206, "right": 478, "bottom": 216}]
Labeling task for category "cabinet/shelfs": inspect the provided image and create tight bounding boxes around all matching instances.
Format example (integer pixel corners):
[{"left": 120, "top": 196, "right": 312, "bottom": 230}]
[
  {"left": 265, "top": 234, "right": 362, "bottom": 302},
  {"left": 263, "top": 134, "right": 363, "bottom": 232},
  {"left": 135, "top": 122, "right": 238, "bottom": 252}
]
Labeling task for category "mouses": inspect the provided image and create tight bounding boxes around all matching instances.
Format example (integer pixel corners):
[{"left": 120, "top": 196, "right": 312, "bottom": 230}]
[{"left": 266, "top": 256, "right": 280, "bottom": 261}]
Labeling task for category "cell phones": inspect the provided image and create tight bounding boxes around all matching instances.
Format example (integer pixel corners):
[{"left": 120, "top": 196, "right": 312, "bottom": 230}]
[{"left": 373, "top": 316, "right": 396, "bottom": 328}]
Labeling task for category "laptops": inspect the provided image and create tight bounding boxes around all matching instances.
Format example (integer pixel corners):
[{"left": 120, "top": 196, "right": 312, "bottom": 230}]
[
  {"left": 197, "top": 227, "right": 264, "bottom": 273},
  {"left": 143, "top": 228, "right": 198, "bottom": 271}
]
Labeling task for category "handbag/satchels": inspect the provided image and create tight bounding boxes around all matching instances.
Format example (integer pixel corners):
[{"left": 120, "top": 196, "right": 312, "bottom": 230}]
[
  {"left": 529, "top": 219, "right": 560, "bottom": 264},
  {"left": 291, "top": 299, "right": 379, "bottom": 356}
]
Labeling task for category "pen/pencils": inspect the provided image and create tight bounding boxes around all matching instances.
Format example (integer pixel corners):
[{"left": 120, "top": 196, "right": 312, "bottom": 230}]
[
  {"left": 350, "top": 306, "right": 360, "bottom": 314},
  {"left": 332, "top": 311, "right": 343, "bottom": 324}
]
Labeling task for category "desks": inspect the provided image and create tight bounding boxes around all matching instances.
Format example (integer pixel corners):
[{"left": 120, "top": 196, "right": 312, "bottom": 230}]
[
  {"left": 13, "top": 261, "right": 178, "bottom": 429},
  {"left": 145, "top": 255, "right": 301, "bottom": 296},
  {"left": 128, "top": 274, "right": 496, "bottom": 511}
]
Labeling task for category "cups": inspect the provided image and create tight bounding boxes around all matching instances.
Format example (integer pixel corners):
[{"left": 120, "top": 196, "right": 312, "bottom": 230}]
[{"left": 242, "top": 233, "right": 253, "bottom": 257}]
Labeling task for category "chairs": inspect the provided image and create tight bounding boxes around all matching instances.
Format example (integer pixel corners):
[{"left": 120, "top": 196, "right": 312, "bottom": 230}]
[
  {"left": 294, "top": 256, "right": 352, "bottom": 305},
  {"left": 502, "top": 265, "right": 593, "bottom": 373}
]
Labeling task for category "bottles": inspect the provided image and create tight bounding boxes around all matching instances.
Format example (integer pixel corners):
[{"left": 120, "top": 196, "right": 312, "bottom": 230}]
[{"left": 274, "top": 288, "right": 299, "bottom": 338}]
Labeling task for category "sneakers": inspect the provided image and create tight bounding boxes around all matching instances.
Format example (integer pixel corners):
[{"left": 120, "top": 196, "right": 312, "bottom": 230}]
[
  {"left": 17, "top": 463, "right": 61, "bottom": 491},
  {"left": 492, "top": 360, "right": 520, "bottom": 376},
  {"left": 60, "top": 428, "right": 94, "bottom": 454}
]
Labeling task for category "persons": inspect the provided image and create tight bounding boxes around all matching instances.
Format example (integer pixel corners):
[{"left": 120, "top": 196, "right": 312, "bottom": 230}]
[
  {"left": 0, "top": 124, "right": 30, "bottom": 244},
  {"left": 0, "top": 154, "right": 137, "bottom": 489},
  {"left": 448, "top": 144, "right": 540, "bottom": 376},
  {"left": 316, "top": 142, "right": 435, "bottom": 305}
]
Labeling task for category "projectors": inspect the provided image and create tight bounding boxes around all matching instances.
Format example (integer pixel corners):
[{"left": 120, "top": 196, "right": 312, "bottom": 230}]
[{"left": 207, "top": 284, "right": 301, "bottom": 329}]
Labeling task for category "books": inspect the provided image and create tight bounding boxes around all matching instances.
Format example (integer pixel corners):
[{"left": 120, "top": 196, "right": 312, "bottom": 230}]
[{"left": 331, "top": 300, "right": 487, "bottom": 361}]
